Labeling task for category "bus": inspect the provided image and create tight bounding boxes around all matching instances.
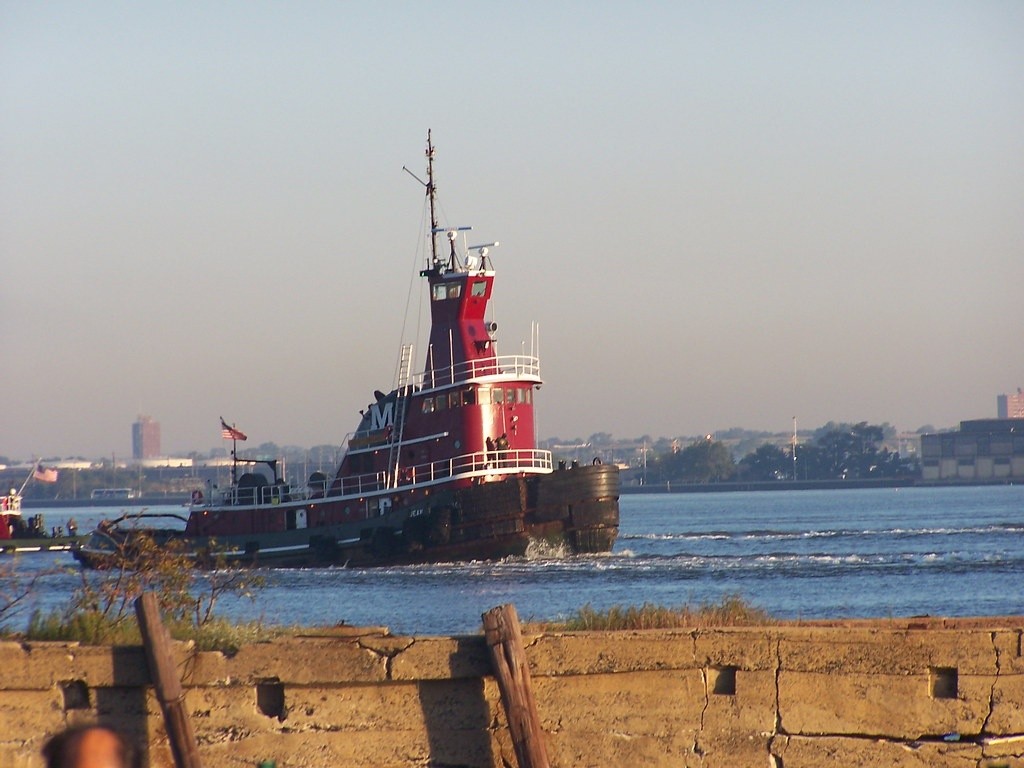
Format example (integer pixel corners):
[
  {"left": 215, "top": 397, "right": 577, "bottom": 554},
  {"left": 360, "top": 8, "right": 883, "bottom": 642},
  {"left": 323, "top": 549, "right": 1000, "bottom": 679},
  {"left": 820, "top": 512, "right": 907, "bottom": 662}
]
[{"left": 90, "top": 488, "right": 134, "bottom": 499}]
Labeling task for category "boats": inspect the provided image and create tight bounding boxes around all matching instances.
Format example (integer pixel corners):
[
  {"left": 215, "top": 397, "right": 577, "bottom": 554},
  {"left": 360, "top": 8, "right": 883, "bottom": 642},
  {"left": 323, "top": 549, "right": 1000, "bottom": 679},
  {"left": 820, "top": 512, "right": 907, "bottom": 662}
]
[
  {"left": 0, "top": 488, "right": 93, "bottom": 552},
  {"left": 73, "top": 128, "right": 621, "bottom": 571}
]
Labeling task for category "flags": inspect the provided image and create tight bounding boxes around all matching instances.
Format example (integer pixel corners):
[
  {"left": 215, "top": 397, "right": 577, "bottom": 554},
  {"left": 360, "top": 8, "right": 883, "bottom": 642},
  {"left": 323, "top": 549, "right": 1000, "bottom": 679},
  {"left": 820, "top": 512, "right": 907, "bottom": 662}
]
[
  {"left": 222, "top": 422, "right": 247, "bottom": 441},
  {"left": 34, "top": 465, "right": 58, "bottom": 482}
]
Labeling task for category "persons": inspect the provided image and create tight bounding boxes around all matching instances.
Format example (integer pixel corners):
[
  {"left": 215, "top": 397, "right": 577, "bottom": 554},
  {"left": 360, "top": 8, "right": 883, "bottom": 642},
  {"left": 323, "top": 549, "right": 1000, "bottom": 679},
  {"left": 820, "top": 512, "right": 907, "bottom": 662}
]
[
  {"left": 495, "top": 434, "right": 511, "bottom": 467},
  {"left": 486, "top": 437, "right": 496, "bottom": 467}
]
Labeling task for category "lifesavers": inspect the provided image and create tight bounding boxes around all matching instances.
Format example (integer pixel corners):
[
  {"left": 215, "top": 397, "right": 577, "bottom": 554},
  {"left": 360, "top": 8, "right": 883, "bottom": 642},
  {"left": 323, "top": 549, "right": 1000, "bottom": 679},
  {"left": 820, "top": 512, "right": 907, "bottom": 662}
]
[
  {"left": 192, "top": 490, "right": 204, "bottom": 504},
  {"left": 397, "top": 466, "right": 412, "bottom": 486}
]
